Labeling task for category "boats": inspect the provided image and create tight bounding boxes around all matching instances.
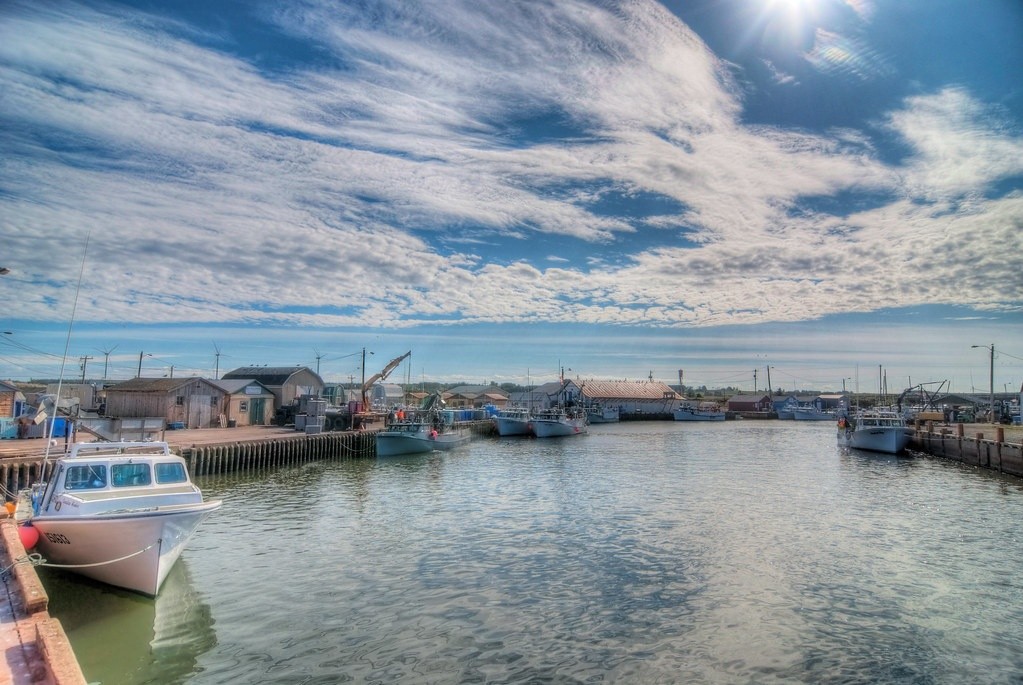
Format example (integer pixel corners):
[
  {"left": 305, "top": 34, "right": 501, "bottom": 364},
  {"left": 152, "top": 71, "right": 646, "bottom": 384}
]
[
  {"left": 374, "top": 408, "right": 472, "bottom": 457},
  {"left": 492, "top": 357, "right": 620, "bottom": 438},
  {"left": 14, "top": 231, "right": 223, "bottom": 602},
  {"left": 672, "top": 402, "right": 726, "bottom": 422},
  {"left": 775, "top": 379, "right": 943, "bottom": 422},
  {"left": 837, "top": 362, "right": 917, "bottom": 454}
]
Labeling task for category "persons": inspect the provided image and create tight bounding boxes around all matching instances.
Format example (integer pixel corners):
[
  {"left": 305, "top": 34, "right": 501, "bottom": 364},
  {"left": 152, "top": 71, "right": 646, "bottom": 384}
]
[
  {"left": 396, "top": 408, "right": 404, "bottom": 422},
  {"left": 388, "top": 411, "right": 395, "bottom": 424}
]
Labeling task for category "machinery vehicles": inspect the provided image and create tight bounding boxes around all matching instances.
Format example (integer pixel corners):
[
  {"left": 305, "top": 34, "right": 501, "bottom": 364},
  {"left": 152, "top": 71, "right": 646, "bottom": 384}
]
[{"left": 353, "top": 349, "right": 411, "bottom": 423}]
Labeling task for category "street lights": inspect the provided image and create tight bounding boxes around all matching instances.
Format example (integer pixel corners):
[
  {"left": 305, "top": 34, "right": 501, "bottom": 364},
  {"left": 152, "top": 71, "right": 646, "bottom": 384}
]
[
  {"left": 361, "top": 346, "right": 375, "bottom": 385},
  {"left": 970, "top": 344, "right": 995, "bottom": 425},
  {"left": 137, "top": 351, "right": 154, "bottom": 379},
  {"left": 1003, "top": 382, "right": 1012, "bottom": 401},
  {"left": 767, "top": 365, "right": 775, "bottom": 413},
  {"left": 843, "top": 377, "right": 852, "bottom": 391}
]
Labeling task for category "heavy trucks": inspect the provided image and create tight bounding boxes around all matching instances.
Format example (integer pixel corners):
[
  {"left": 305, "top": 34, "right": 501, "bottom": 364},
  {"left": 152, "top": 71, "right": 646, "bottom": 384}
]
[{"left": 270, "top": 395, "right": 364, "bottom": 432}]
